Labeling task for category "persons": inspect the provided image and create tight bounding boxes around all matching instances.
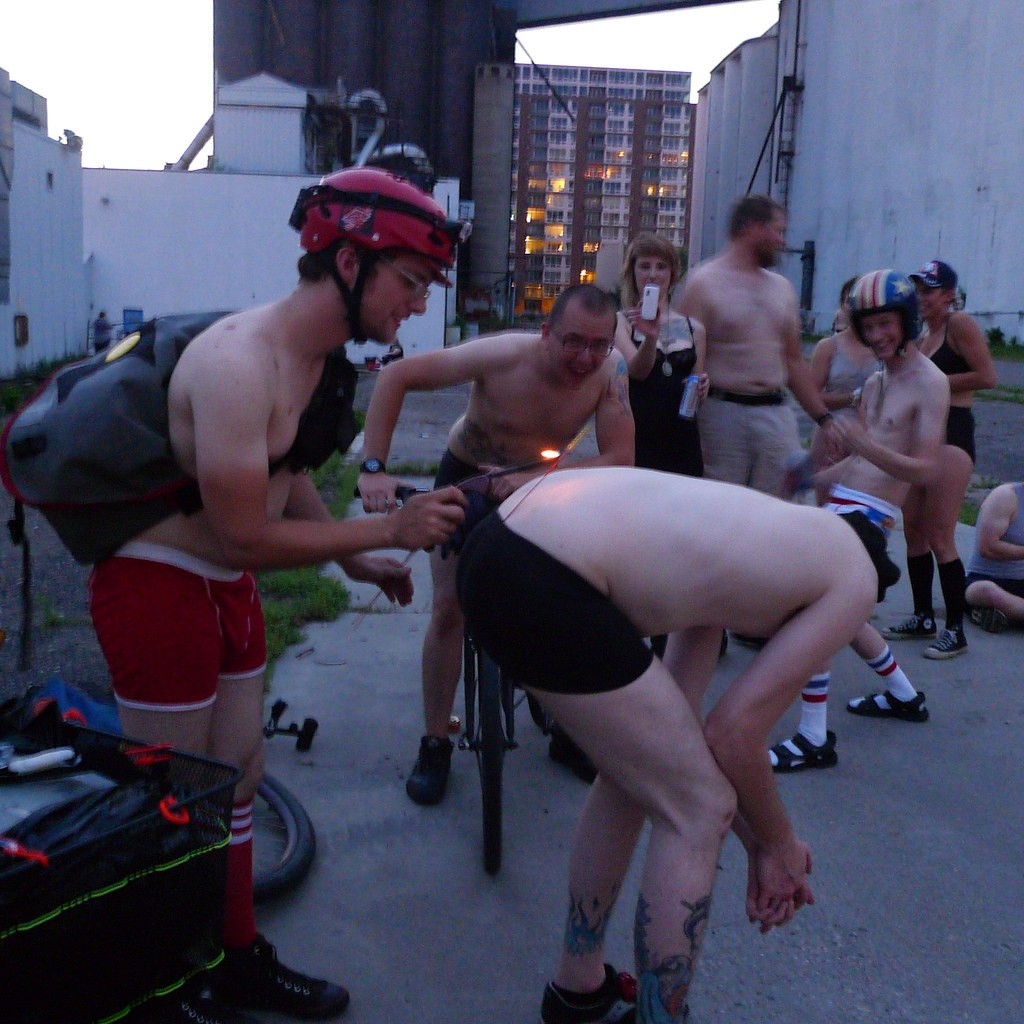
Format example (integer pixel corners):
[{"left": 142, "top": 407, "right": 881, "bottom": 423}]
[
  {"left": 92, "top": 311, "right": 113, "bottom": 355},
  {"left": 808, "top": 276, "right": 883, "bottom": 515},
  {"left": 673, "top": 195, "right": 842, "bottom": 647},
  {"left": 766, "top": 268, "right": 928, "bottom": 774},
  {"left": 963, "top": 480, "right": 1024, "bottom": 636},
  {"left": 83, "top": 165, "right": 469, "bottom": 1024},
  {"left": 880, "top": 260, "right": 999, "bottom": 660},
  {"left": 376, "top": 337, "right": 403, "bottom": 371},
  {"left": 456, "top": 463, "right": 901, "bottom": 1024},
  {"left": 615, "top": 229, "right": 731, "bottom": 667},
  {"left": 357, "top": 282, "right": 635, "bottom": 806}
]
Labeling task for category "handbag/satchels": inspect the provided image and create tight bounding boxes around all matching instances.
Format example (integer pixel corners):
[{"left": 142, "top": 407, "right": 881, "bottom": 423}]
[{"left": 0, "top": 307, "right": 255, "bottom": 565}]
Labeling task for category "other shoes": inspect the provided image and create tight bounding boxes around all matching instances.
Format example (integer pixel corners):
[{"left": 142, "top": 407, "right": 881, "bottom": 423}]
[
  {"left": 964, "top": 606, "right": 1008, "bottom": 631},
  {"left": 408, "top": 736, "right": 453, "bottom": 804},
  {"left": 549, "top": 727, "right": 599, "bottom": 783}
]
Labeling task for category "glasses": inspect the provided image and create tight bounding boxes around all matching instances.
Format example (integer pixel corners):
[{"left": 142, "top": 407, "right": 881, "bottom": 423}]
[
  {"left": 550, "top": 325, "right": 614, "bottom": 358},
  {"left": 378, "top": 254, "right": 431, "bottom": 301}
]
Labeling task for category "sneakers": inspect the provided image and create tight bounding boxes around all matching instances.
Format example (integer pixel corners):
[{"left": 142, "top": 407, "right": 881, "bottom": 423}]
[
  {"left": 542, "top": 963, "right": 638, "bottom": 1024},
  {"left": 924, "top": 630, "right": 968, "bottom": 659},
  {"left": 227, "top": 934, "right": 350, "bottom": 1019},
  {"left": 881, "top": 609, "right": 937, "bottom": 638}
]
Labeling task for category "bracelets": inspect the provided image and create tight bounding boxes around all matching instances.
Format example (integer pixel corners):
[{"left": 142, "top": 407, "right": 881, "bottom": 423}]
[
  {"left": 853, "top": 387, "right": 862, "bottom": 407},
  {"left": 816, "top": 413, "right": 833, "bottom": 428}
]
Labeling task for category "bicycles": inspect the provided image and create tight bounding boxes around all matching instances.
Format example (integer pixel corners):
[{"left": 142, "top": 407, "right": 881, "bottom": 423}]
[
  {"left": 457, "top": 637, "right": 520, "bottom": 878},
  {"left": 241, "top": 694, "right": 318, "bottom": 906}
]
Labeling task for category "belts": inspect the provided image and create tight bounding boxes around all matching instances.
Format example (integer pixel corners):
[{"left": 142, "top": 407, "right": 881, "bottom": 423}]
[{"left": 707, "top": 387, "right": 787, "bottom": 406}]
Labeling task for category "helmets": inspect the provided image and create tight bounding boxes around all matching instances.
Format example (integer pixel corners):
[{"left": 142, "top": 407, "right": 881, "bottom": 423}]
[
  {"left": 290, "top": 168, "right": 473, "bottom": 266},
  {"left": 848, "top": 271, "right": 923, "bottom": 346}
]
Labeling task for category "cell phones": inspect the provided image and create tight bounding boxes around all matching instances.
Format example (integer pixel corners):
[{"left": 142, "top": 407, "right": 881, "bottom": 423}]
[{"left": 642, "top": 287, "right": 660, "bottom": 320}]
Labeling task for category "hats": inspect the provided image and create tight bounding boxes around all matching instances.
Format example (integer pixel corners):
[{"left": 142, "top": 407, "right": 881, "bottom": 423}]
[
  {"left": 908, "top": 261, "right": 957, "bottom": 291},
  {"left": 838, "top": 510, "right": 901, "bottom": 603},
  {"left": 412, "top": 251, "right": 452, "bottom": 290}
]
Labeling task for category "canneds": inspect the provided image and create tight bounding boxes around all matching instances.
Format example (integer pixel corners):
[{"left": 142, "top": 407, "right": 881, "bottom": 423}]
[{"left": 678, "top": 376, "right": 702, "bottom": 419}]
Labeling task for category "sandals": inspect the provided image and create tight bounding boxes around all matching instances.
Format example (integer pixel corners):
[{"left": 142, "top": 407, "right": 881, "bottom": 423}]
[
  {"left": 847, "top": 690, "right": 928, "bottom": 722},
  {"left": 767, "top": 731, "right": 838, "bottom": 771}
]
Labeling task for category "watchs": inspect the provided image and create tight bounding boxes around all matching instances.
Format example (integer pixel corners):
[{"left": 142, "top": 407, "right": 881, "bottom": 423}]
[{"left": 359, "top": 456, "right": 388, "bottom": 474}]
